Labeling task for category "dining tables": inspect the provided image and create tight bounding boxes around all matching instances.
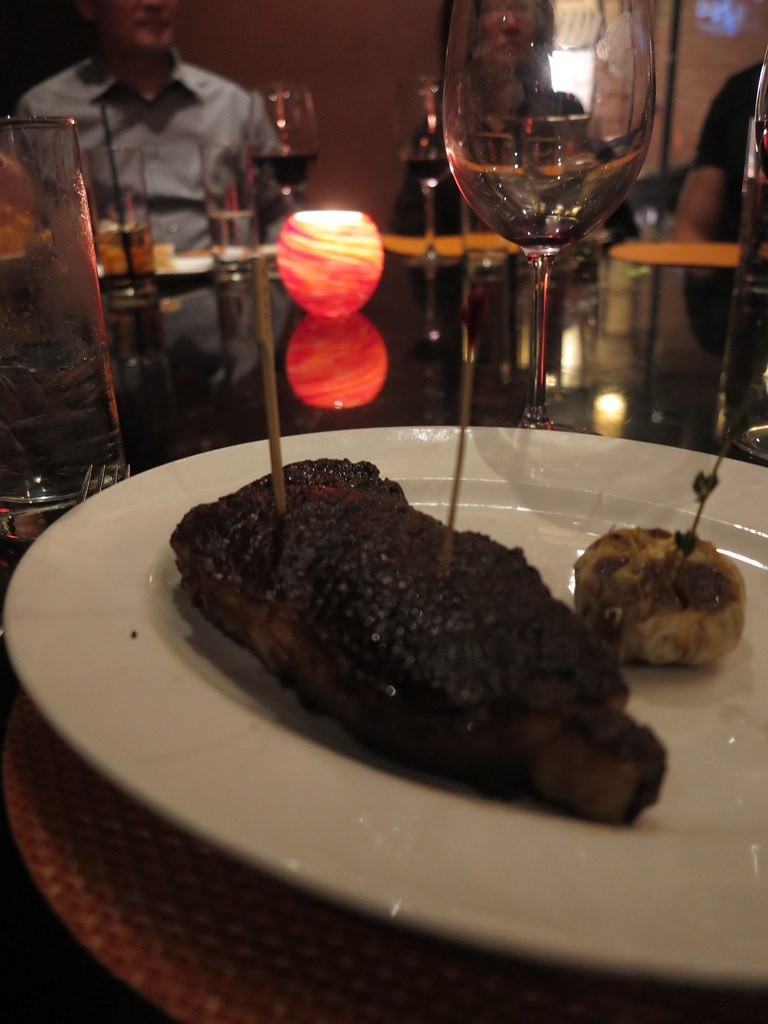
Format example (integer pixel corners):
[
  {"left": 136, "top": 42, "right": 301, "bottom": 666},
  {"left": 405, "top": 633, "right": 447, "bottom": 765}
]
[{"left": 0, "top": 231, "right": 768, "bottom": 1024}]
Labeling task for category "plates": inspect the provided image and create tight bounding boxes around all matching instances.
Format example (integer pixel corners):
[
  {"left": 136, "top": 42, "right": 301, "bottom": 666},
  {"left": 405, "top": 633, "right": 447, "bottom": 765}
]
[{"left": 4, "top": 424, "right": 768, "bottom": 989}]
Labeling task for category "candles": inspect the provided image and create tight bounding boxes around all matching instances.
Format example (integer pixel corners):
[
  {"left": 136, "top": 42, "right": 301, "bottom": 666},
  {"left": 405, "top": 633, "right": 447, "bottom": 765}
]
[
  {"left": 275, "top": 209, "right": 385, "bottom": 319},
  {"left": 285, "top": 311, "right": 388, "bottom": 409}
]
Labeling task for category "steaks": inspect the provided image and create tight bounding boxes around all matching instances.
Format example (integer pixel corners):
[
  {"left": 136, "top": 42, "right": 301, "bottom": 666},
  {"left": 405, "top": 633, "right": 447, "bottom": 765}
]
[{"left": 168, "top": 454, "right": 667, "bottom": 826}]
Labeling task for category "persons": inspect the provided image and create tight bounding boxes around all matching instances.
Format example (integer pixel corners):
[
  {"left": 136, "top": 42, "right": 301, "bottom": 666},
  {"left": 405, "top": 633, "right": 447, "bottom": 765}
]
[
  {"left": 674, "top": 60, "right": 768, "bottom": 372},
  {"left": 388, "top": 0, "right": 640, "bottom": 376},
  {"left": 0, "top": 0, "right": 290, "bottom": 472}
]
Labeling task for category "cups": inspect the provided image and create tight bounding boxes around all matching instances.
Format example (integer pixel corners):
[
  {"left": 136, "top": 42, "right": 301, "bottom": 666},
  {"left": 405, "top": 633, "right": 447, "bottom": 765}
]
[
  {"left": 0, "top": 116, "right": 130, "bottom": 542},
  {"left": 79, "top": 146, "right": 161, "bottom": 310},
  {"left": 198, "top": 141, "right": 262, "bottom": 285}
]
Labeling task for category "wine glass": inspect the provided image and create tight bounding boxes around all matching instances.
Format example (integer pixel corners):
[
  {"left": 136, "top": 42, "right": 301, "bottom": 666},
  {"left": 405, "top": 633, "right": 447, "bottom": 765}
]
[
  {"left": 439, "top": 0, "right": 658, "bottom": 441},
  {"left": 724, "top": 48, "right": 768, "bottom": 462},
  {"left": 397, "top": 74, "right": 458, "bottom": 268},
  {"left": 246, "top": 82, "right": 318, "bottom": 218}
]
